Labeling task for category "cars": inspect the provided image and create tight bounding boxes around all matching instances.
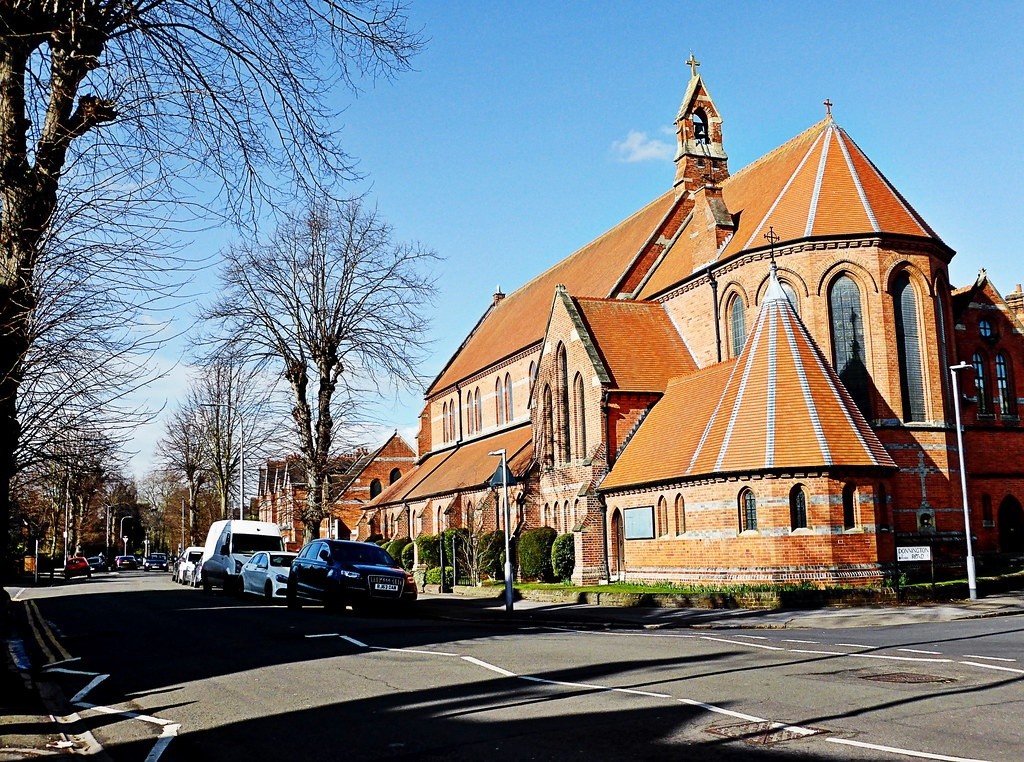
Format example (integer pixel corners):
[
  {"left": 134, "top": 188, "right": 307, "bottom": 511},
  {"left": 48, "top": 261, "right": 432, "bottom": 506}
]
[
  {"left": 142, "top": 553, "right": 168, "bottom": 572},
  {"left": 171, "top": 547, "right": 204, "bottom": 585},
  {"left": 288, "top": 539, "right": 418, "bottom": 614},
  {"left": 64, "top": 557, "right": 91, "bottom": 584},
  {"left": 87, "top": 557, "right": 106, "bottom": 573},
  {"left": 236, "top": 551, "right": 299, "bottom": 604},
  {"left": 116, "top": 556, "right": 137, "bottom": 571}
]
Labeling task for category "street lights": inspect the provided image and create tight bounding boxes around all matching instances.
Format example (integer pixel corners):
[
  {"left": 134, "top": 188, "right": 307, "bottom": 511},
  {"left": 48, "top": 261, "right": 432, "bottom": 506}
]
[
  {"left": 488, "top": 448, "right": 514, "bottom": 614},
  {"left": 62, "top": 471, "right": 94, "bottom": 567},
  {"left": 119, "top": 515, "right": 133, "bottom": 539},
  {"left": 949, "top": 360, "right": 979, "bottom": 602}
]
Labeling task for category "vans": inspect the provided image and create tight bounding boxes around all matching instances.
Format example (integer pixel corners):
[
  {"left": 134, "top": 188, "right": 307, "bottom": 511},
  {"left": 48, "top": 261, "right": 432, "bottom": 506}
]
[{"left": 200, "top": 519, "right": 286, "bottom": 592}]
